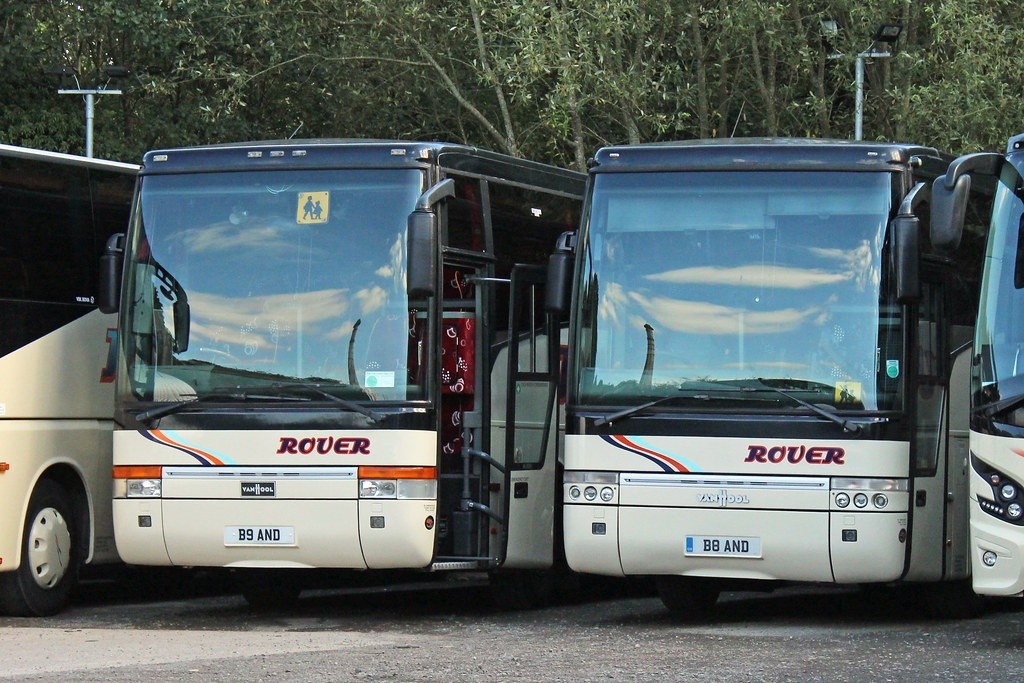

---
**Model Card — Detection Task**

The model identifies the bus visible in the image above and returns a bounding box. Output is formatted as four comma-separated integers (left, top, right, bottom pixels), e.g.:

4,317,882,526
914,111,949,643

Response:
546,136,1002,590
0,144,141,621
92,137,589,569
930,134,1024,597
